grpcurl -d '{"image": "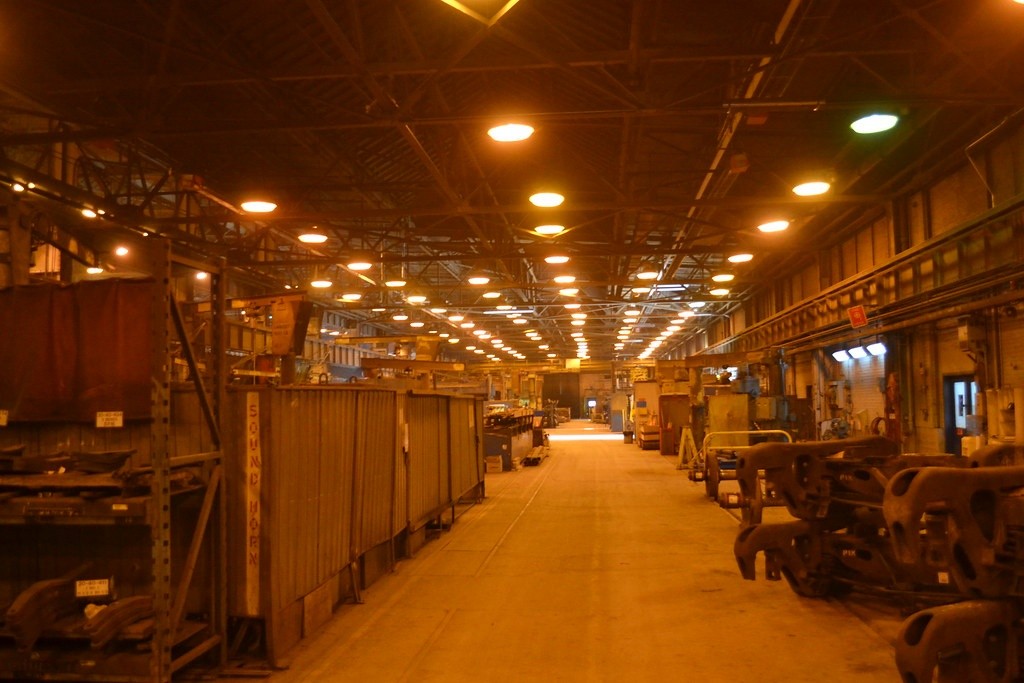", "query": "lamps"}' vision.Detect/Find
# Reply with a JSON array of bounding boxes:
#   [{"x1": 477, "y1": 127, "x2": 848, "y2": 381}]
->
[
  {"x1": 614, "y1": 267, "x2": 707, "y2": 361},
  {"x1": 242, "y1": 192, "x2": 278, "y2": 213},
  {"x1": 833, "y1": 335, "x2": 889, "y2": 362},
  {"x1": 792, "y1": 164, "x2": 830, "y2": 197},
  {"x1": 710, "y1": 285, "x2": 731, "y2": 297},
  {"x1": 298, "y1": 223, "x2": 327, "y2": 244},
  {"x1": 310, "y1": 265, "x2": 333, "y2": 287},
  {"x1": 468, "y1": 272, "x2": 492, "y2": 285},
  {"x1": 728, "y1": 251, "x2": 753, "y2": 264},
  {"x1": 348, "y1": 262, "x2": 408, "y2": 287},
  {"x1": 534, "y1": 216, "x2": 566, "y2": 234},
  {"x1": 545, "y1": 250, "x2": 571, "y2": 264},
  {"x1": 486, "y1": 106, "x2": 538, "y2": 143},
  {"x1": 528, "y1": 179, "x2": 564, "y2": 209},
  {"x1": 663, "y1": 358, "x2": 665, "y2": 362},
  {"x1": 555, "y1": 269, "x2": 576, "y2": 282},
  {"x1": 757, "y1": 207, "x2": 791, "y2": 233},
  {"x1": 334, "y1": 290, "x2": 591, "y2": 360},
  {"x1": 711, "y1": 270, "x2": 736, "y2": 283},
  {"x1": 851, "y1": 86, "x2": 898, "y2": 135}
]
